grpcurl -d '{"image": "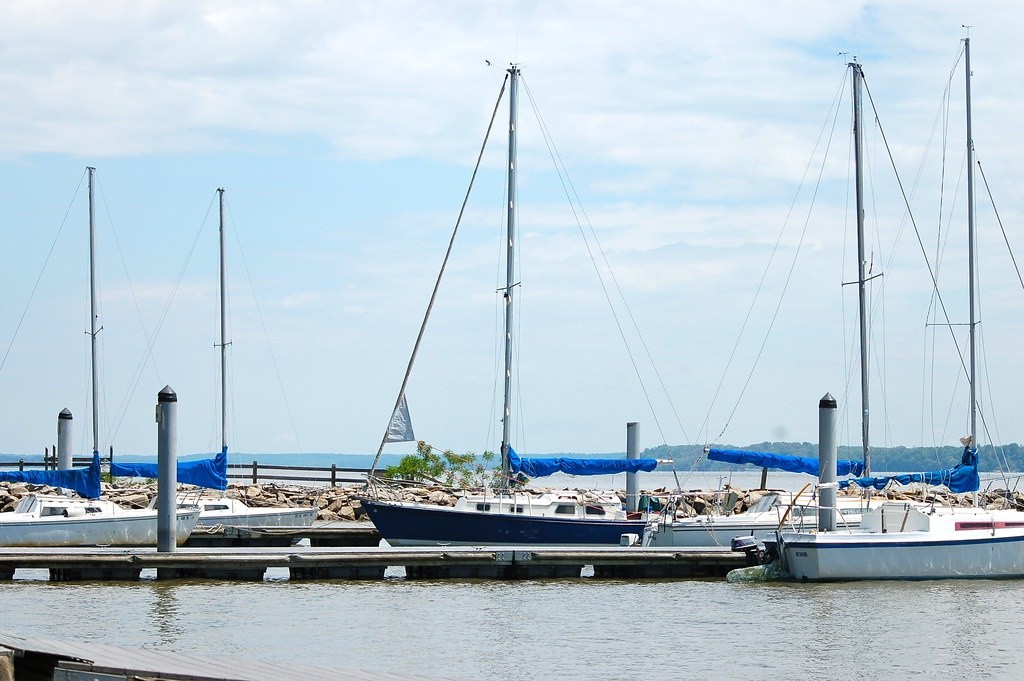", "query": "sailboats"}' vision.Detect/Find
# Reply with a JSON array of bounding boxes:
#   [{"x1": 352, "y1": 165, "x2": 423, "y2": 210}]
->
[
  {"x1": 356, "y1": 55, "x2": 717, "y2": 548},
  {"x1": 137, "y1": 186, "x2": 321, "y2": 548},
  {"x1": 631, "y1": 50, "x2": 1024, "y2": 548},
  {"x1": 0, "y1": 164, "x2": 204, "y2": 547},
  {"x1": 771, "y1": 25, "x2": 1024, "y2": 582}
]
